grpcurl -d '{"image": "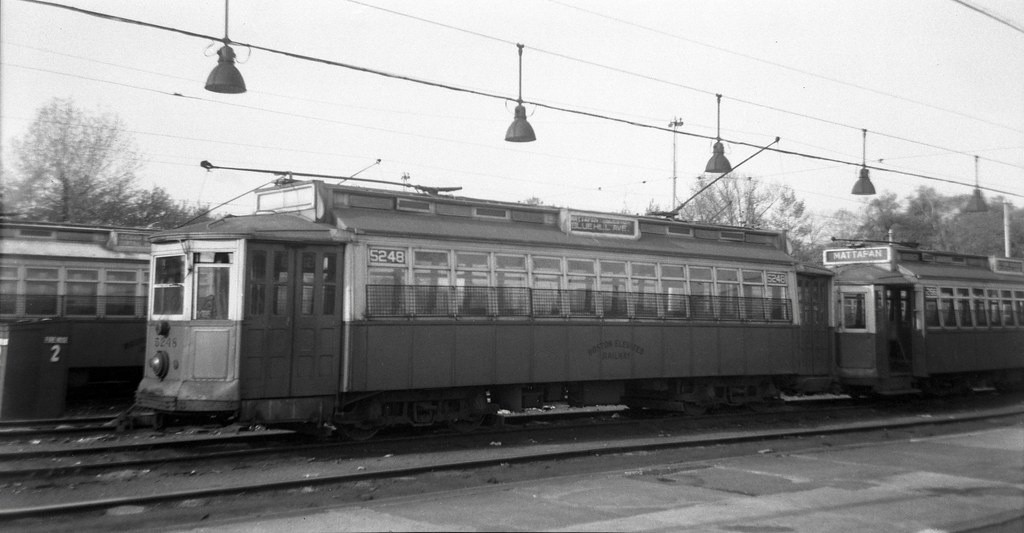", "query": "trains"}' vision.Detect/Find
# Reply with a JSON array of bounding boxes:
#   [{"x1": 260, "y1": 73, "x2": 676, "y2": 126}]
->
[
  {"x1": 0, "y1": 216, "x2": 153, "y2": 394},
  {"x1": 132, "y1": 179, "x2": 1022, "y2": 442}
]
